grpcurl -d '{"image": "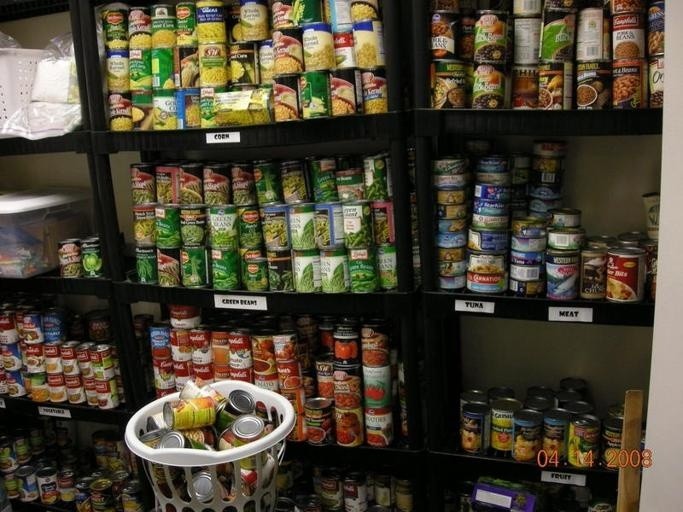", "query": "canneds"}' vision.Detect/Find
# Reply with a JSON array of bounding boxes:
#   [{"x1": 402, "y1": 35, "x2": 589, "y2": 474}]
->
[{"x1": 0, "y1": 0, "x2": 664, "y2": 512}]
